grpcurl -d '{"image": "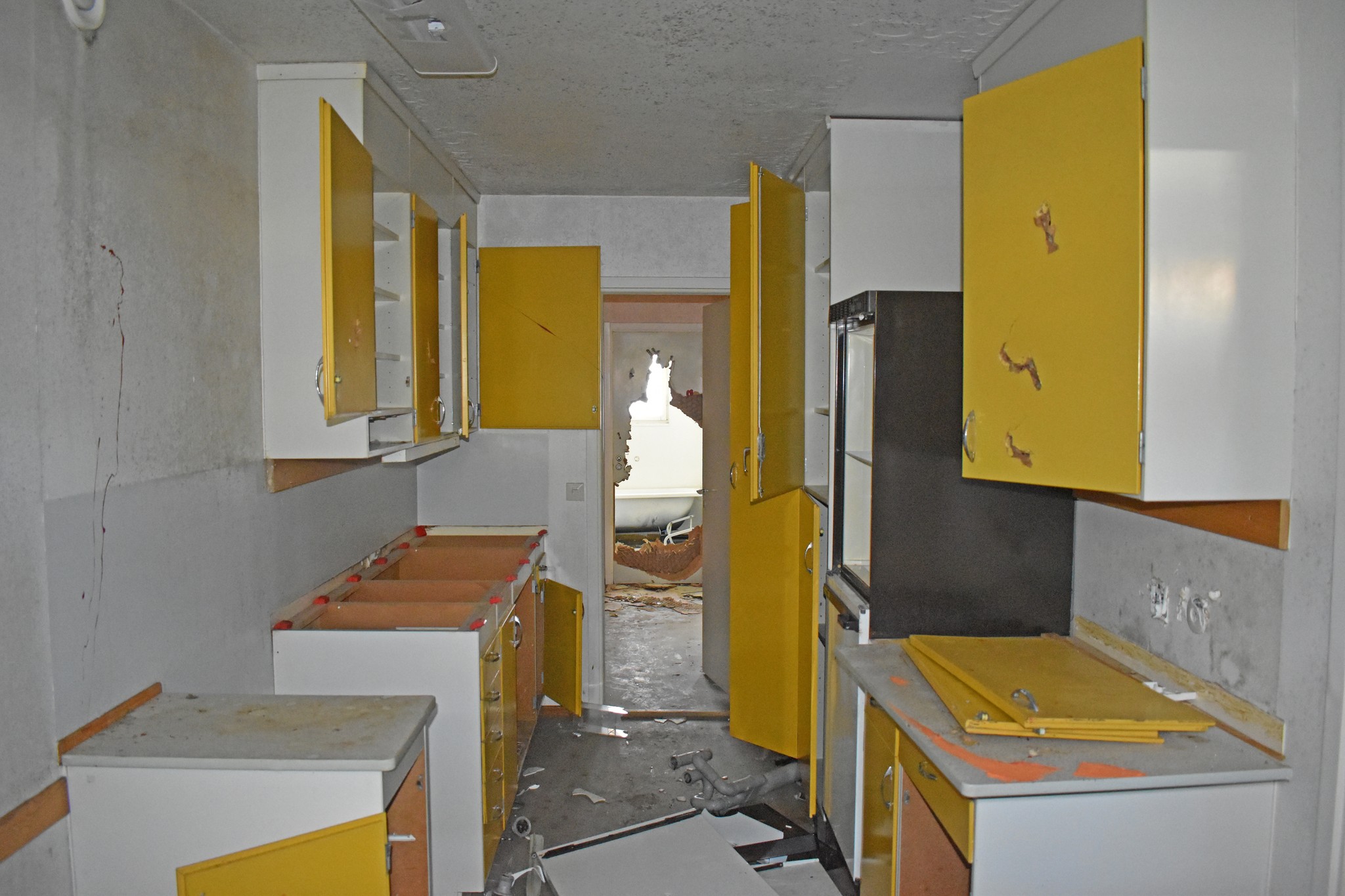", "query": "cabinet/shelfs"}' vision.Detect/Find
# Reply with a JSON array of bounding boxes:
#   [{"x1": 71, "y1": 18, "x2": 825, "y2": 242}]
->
[
  {"x1": 252, "y1": 69, "x2": 603, "y2": 466},
  {"x1": 728, "y1": 36, "x2": 1299, "y2": 896},
  {"x1": 62, "y1": 525, "x2": 584, "y2": 896}
]
